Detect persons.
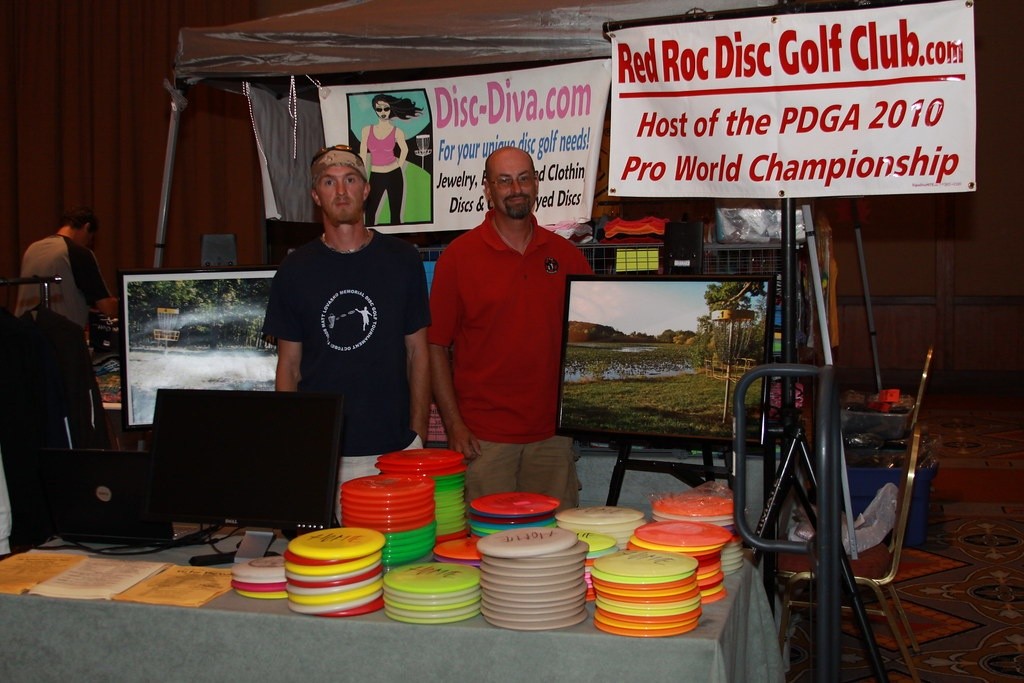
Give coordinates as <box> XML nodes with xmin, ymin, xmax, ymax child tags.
<box><xmin>14</xmin><ymin>205</ymin><xmax>118</xmax><ymax>331</ymax></box>
<box><xmin>431</xmin><ymin>146</ymin><xmax>596</xmax><ymax>508</ymax></box>
<box><xmin>263</xmin><ymin>145</ymin><xmax>432</xmax><ymax>523</ymax></box>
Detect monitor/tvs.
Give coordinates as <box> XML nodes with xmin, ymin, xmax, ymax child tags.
<box><xmin>149</xmin><ymin>389</ymin><xmax>344</xmax><ymax>566</ymax></box>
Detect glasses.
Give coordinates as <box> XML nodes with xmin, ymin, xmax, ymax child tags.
<box><xmin>311</xmin><ymin>145</ymin><xmax>362</xmax><ymax>164</ymax></box>
<box><xmin>489</xmin><ymin>174</ymin><xmax>535</xmax><ymax>186</ymax></box>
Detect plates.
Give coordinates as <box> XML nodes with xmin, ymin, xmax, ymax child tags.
<box><xmin>231</xmin><ymin>449</ymin><xmax>745</xmax><ymax>638</ymax></box>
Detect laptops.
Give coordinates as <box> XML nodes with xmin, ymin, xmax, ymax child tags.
<box><xmin>37</xmin><ymin>447</ymin><xmax>219</xmax><ymax>547</ymax></box>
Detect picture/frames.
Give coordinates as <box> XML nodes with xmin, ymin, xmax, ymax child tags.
<box><xmin>556</xmin><ymin>273</ymin><xmax>777</xmax><ymax>448</ymax></box>
<box><xmin>117</xmin><ymin>265</ymin><xmax>278</xmax><ymax>432</ymax></box>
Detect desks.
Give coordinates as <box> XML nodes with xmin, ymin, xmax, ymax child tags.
<box><xmin>0</xmin><ymin>521</ymin><xmax>800</xmax><ymax>683</ymax></box>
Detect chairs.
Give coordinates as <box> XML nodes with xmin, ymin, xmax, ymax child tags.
<box><xmin>776</xmin><ymin>423</ymin><xmax>921</xmax><ymax>683</ymax></box>
<box><xmin>887</xmin><ymin>345</ymin><xmax>934</xmax><ymax>446</ymax></box>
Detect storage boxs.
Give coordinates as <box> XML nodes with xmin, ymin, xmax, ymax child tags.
<box><xmin>714</xmin><ymin>197</ymin><xmax>806</xmax><ymax>243</ymax></box>
<box><xmin>845</xmin><ymin>449</ymin><xmax>935</xmax><ymax>547</ymax></box>
<box><xmin>840</xmin><ymin>394</ymin><xmax>915</xmax><ymax>439</ymax></box>
